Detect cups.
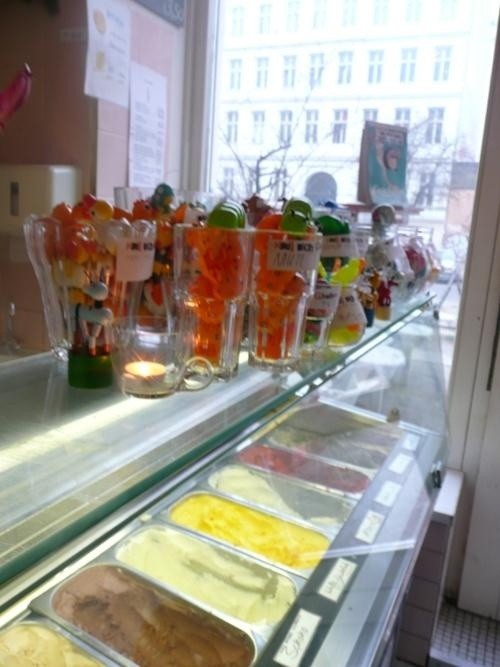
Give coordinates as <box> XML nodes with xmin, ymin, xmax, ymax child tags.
<box><xmin>20</xmin><ymin>214</ymin><xmax>367</xmax><ymax>399</ymax></box>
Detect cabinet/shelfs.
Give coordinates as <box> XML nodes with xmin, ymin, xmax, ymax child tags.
<box><xmin>0</xmin><ymin>289</ymin><xmax>453</xmax><ymax>667</ymax></box>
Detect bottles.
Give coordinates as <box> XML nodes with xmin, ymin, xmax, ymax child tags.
<box><xmin>353</xmin><ymin>223</ymin><xmax>443</xmax><ymax>308</ymax></box>
